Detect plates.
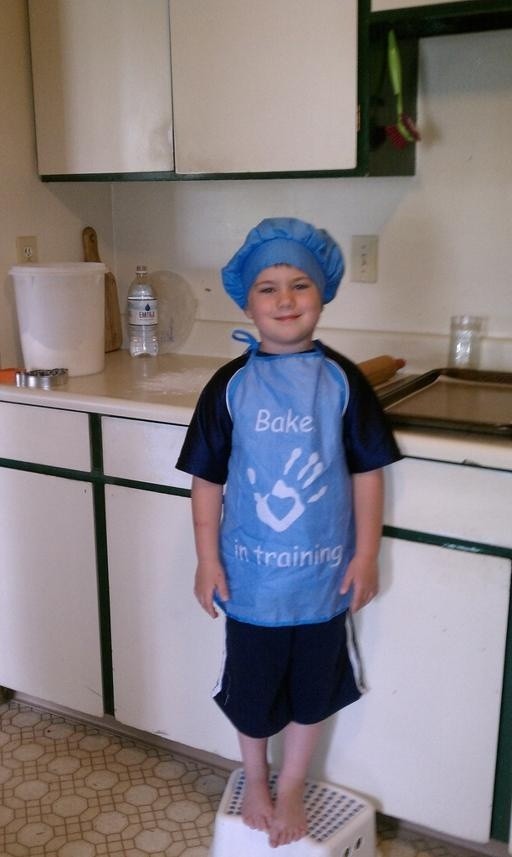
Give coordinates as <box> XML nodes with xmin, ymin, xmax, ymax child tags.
<box><xmin>379</xmin><ymin>366</ymin><xmax>511</xmax><ymax>438</ymax></box>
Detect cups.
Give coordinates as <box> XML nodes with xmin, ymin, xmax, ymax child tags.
<box><xmin>449</xmin><ymin>315</ymin><xmax>484</xmax><ymax>372</ymax></box>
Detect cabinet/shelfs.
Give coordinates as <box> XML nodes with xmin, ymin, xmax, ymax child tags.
<box><xmin>91</xmin><ymin>414</ymin><xmax>510</xmax><ymax>856</ymax></box>
<box><xmin>0</xmin><ymin>398</ymin><xmax>106</xmax><ymax>731</ymax></box>
<box><xmin>22</xmin><ymin>0</ymin><xmax>416</xmax><ymax>183</ymax></box>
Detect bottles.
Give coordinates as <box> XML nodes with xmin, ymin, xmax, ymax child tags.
<box><xmin>126</xmin><ymin>265</ymin><xmax>159</xmax><ymax>360</ymax></box>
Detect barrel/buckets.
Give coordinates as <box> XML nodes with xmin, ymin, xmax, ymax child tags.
<box><xmin>7</xmin><ymin>260</ymin><xmax>110</xmax><ymax>377</ymax></box>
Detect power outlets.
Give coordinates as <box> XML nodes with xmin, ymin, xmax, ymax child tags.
<box><xmin>349</xmin><ymin>234</ymin><xmax>378</xmax><ymax>285</ymax></box>
<box><xmin>15</xmin><ymin>235</ymin><xmax>39</xmax><ymax>266</ymax></box>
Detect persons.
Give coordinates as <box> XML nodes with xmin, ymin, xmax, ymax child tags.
<box><xmin>175</xmin><ymin>215</ymin><xmax>405</xmax><ymax>847</ymax></box>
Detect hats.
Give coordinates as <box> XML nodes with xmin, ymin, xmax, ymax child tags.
<box><xmin>219</xmin><ymin>216</ymin><xmax>347</xmax><ymax>311</ymax></box>
